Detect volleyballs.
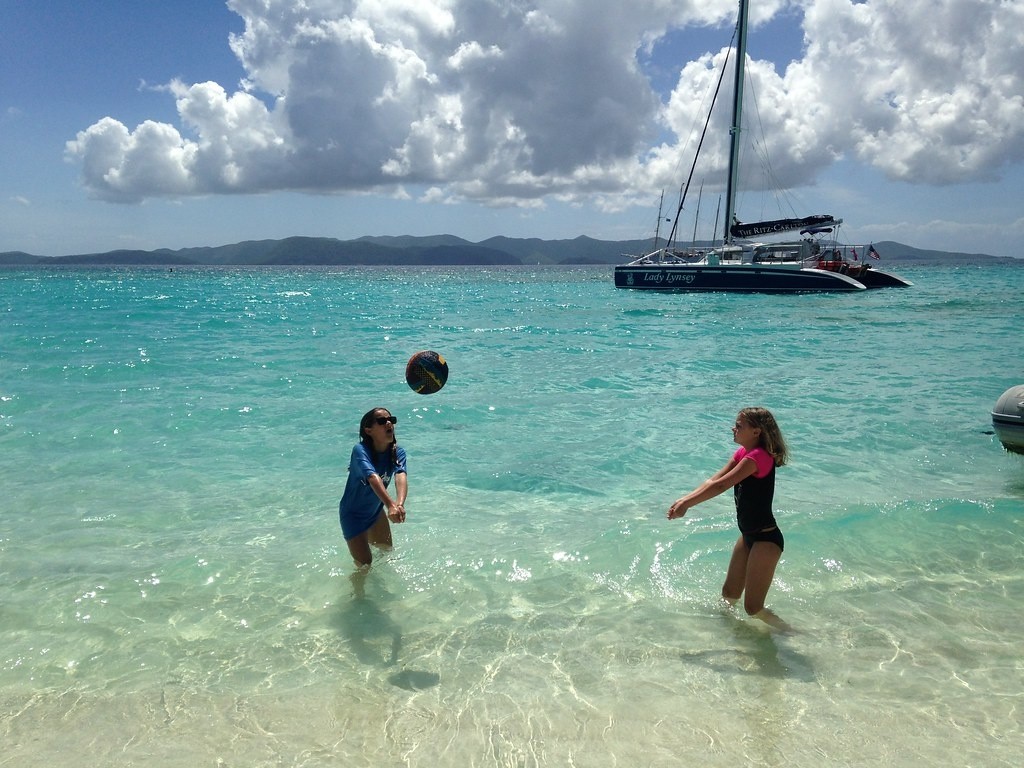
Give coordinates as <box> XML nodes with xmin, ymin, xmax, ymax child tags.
<box><xmin>404</xmin><ymin>350</ymin><xmax>449</xmax><ymax>395</ymax></box>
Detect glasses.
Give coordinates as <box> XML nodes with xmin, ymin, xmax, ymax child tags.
<box><xmin>375</xmin><ymin>417</ymin><xmax>396</xmax><ymax>425</ymax></box>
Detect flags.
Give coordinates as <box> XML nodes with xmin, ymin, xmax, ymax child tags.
<box><xmin>866</xmin><ymin>243</ymin><xmax>880</xmax><ymax>260</ymax></box>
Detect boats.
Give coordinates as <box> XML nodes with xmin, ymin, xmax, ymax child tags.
<box><xmin>817</xmin><ymin>261</ymin><xmax>871</xmax><ymax>277</ymax></box>
<box><xmin>992</xmin><ymin>385</ymin><xmax>1024</xmax><ymax>455</ymax></box>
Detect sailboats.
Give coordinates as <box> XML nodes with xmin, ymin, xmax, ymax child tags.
<box><xmin>613</xmin><ymin>1</ymin><xmax>914</xmax><ymax>293</ymax></box>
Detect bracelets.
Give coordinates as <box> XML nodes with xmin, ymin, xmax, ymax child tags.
<box><xmin>397</xmin><ymin>505</ymin><xmax>404</xmax><ymax>508</ymax></box>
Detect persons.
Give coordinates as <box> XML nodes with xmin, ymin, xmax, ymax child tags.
<box><xmin>666</xmin><ymin>407</ymin><xmax>790</xmax><ymax>615</ymax></box>
<box><xmin>850</xmin><ymin>249</ymin><xmax>858</xmax><ymax>261</ymax></box>
<box><xmin>339</xmin><ymin>409</ymin><xmax>408</xmax><ymax>565</ymax></box>
